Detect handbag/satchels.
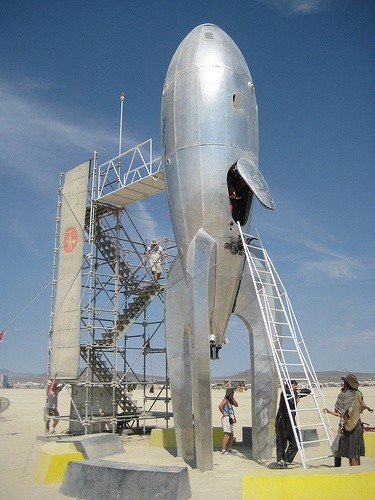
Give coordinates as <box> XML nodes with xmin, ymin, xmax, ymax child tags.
<box><xmin>229</xmin><ymin>414</ymin><xmax>236</xmax><ymax>423</ymax></box>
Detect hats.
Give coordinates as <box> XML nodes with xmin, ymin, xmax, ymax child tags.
<box><xmin>285</xmin><ymin>380</ymin><xmax>297</xmax><ymax>387</ymax></box>
<box><xmin>341</xmin><ymin>374</ymin><xmax>359</xmax><ymax>388</ymax></box>
<box><xmin>225</xmin><ymin>387</ymin><xmax>237</xmax><ymax>397</ymax></box>
<box><xmin>150</xmin><ymin>239</ymin><xmax>159</xmax><ymax>244</ymax></box>
<box><xmin>47</xmin><ymin>380</ymin><xmax>58</xmax><ymax>386</ymax></box>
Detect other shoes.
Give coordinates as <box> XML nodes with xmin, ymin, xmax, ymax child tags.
<box><xmin>229</xmin><ymin>449</ymin><xmax>238</xmax><ymax>455</ymax></box>
<box><xmin>51</xmin><ymin>429</ymin><xmax>58</xmax><ymax>435</ymax></box>
<box><xmin>44</xmin><ymin>433</ymin><xmax>50</xmax><ymax>435</ymax></box>
<box><xmin>277</xmin><ymin>459</ymin><xmax>288</xmax><ymax>469</ymax></box>
<box><xmin>221</xmin><ymin>450</ymin><xmax>230</xmax><ymax>455</ymax></box>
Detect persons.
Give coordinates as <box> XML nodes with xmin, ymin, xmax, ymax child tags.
<box><xmin>218</xmin><ymin>389</ymin><xmax>238</xmax><ymax>455</ymax></box>
<box><xmin>44</xmin><ymin>372</ymin><xmax>65</xmax><ymax>435</ymax></box>
<box><xmin>147</xmin><ymin>240</ymin><xmax>164</xmax><ymax>283</ymax></box>
<box><xmin>275</xmin><ymin>380</ymin><xmax>312</xmax><ymax>469</ymax></box>
<box><xmin>323</xmin><ymin>374</ymin><xmax>366</xmax><ymax>467</ymax></box>
<box><xmin>228</xmin><ymin>177</ymin><xmax>246</xmax><ymax>214</ymax></box>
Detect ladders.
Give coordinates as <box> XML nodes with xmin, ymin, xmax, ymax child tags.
<box><xmin>237</xmin><ymin>219</ymin><xmax>335</xmax><ymax>472</ymax></box>
